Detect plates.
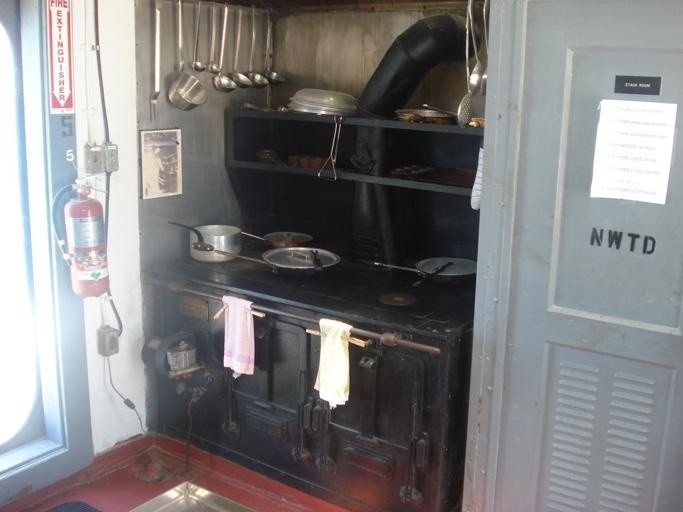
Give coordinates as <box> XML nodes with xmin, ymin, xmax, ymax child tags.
<box><xmin>394</xmin><ymin>109</ymin><xmax>458</xmax><ymax>125</ymax></box>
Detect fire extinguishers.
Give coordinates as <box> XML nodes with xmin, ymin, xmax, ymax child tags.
<box><xmin>51</xmin><ymin>177</ymin><xmax>110</xmax><ymax>298</ymax></box>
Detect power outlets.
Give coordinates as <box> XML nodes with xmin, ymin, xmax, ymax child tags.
<box><xmin>97</xmin><ymin>325</ymin><xmax>122</xmax><ymax>356</ymax></box>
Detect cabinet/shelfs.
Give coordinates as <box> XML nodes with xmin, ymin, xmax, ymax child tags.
<box><xmin>224</xmin><ymin>108</ymin><xmax>484</xmax><ymax>286</ymax></box>
<box><xmin>144</xmin><ymin>257</ymin><xmax>472</xmax><ymax>512</ymax></box>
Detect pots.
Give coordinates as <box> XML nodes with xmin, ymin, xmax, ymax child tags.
<box><xmin>374</xmin><ymin>256</ymin><xmax>477</xmax><ymax>282</ymax></box>
<box><xmin>241</xmin><ymin>231</ymin><xmax>314</xmax><ymax>250</ymax></box>
<box><xmin>168</xmin><ymin>221</ymin><xmax>243</xmax><ymax>264</ymax></box>
<box><xmin>215</xmin><ymin>246</ymin><xmax>342</xmax><ymax>275</ymax></box>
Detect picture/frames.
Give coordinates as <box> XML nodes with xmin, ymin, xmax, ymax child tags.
<box><xmin>140</xmin><ymin>128</ymin><xmax>183</xmax><ymax>200</ymax></box>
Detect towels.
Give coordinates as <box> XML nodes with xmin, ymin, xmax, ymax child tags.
<box><xmin>313</xmin><ymin>318</ymin><xmax>353</xmax><ymax>411</ymax></box>
<box><xmin>221</xmin><ymin>295</ymin><xmax>254</xmax><ymax>379</ymax></box>
